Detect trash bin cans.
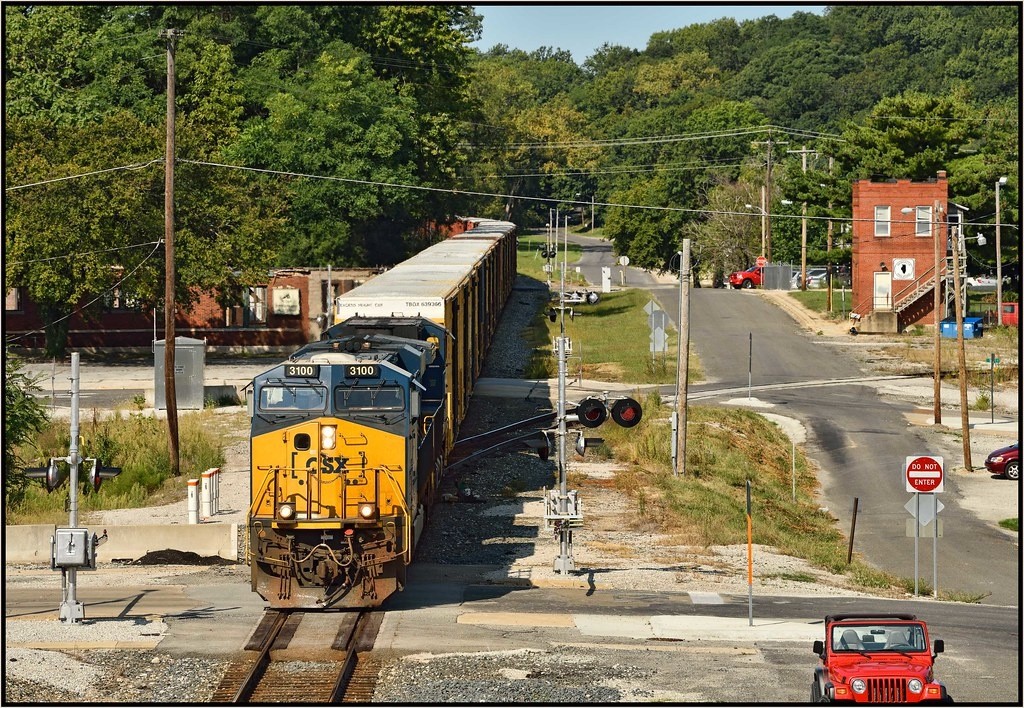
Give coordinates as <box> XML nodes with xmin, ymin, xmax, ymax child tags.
<box><xmin>940</xmin><ymin>316</ymin><xmax>983</xmax><ymax>339</ymax></box>
<box><xmin>601</xmin><ymin>266</ymin><xmax>611</xmax><ymax>293</ymax></box>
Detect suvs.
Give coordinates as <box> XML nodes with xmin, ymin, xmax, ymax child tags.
<box><xmin>985</xmin><ymin>303</ymin><xmax>1018</xmax><ymax>329</ymax></box>
<box><xmin>810</xmin><ymin>612</ymin><xmax>954</xmax><ymax>704</ymax></box>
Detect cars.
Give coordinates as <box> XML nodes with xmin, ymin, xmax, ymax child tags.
<box><xmin>985</xmin><ymin>444</ymin><xmax>1019</xmax><ymax>480</ymax></box>
<box><xmin>731</xmin><ymin>264</ymin><xmax>852</xmax><ymax>289</ymax></box>
<box><xmin>967</xmin><ymin>275</ymin><xmax>1011</xmax><ymax>286</ymax></box>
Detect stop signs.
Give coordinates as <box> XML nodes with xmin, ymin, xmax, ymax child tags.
<box><xmin>756</xmin><ymin>255</ymin><xmax>768</xmax><ymax>266</ymax></box>
<box><xmin>905</xmin><ymin>456</ymin><xmax>943</xmax><ymax>493</ymax></box>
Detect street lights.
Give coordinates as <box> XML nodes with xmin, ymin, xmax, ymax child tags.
<box><xmin>952</xmin><ymin>226</ymin><xmax>987</xmax><ymax>471</ymax></box>
<box><xmin>564</xmin><ymin>215</ymin><xmax>571</xmax><ymax>278</ymax></box>
<box><xmin>746</xmin><ymin>186</ymin><xmax>766</xmax><ymax>256</ymax></box>
<box><xmin>900</xmin><ymin>200</ymin><xmax>942</xmax><ymax>425</ymax></box>
<box><xmin>996</xmin><ymin>177</ymin><xmax>1008</xmax><ymax>326</ymax></box>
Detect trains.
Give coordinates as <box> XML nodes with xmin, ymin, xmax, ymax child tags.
<box><xmin>240</xmin><ymin>215</ymin><xmax>517</xmax><ymax>608</ymax></box>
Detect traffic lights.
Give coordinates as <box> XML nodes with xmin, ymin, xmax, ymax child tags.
<box><xmin>575</xmin><ymin>397</ymin><xmax>643</xmax><ymax>428</ymax></box>
<box><xmin>26</xmin><ymin>458</ymin><xmax>58</xmax><ymax>493</ymax></box>
<box><xmin>88</xmin><ymin>459</ymin><xmax>122</xmax><ymax>493</ymax></box>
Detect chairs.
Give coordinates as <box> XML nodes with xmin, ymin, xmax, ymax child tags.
<box><xmin>836</xmin><ymin>630</ymin><xmax>865</xmax><ymax>650</ymax></box>
<box><xmin>883</xmin><ymin>631</ymin><xmax>910</xmax><ymax>651</ymax></box>
